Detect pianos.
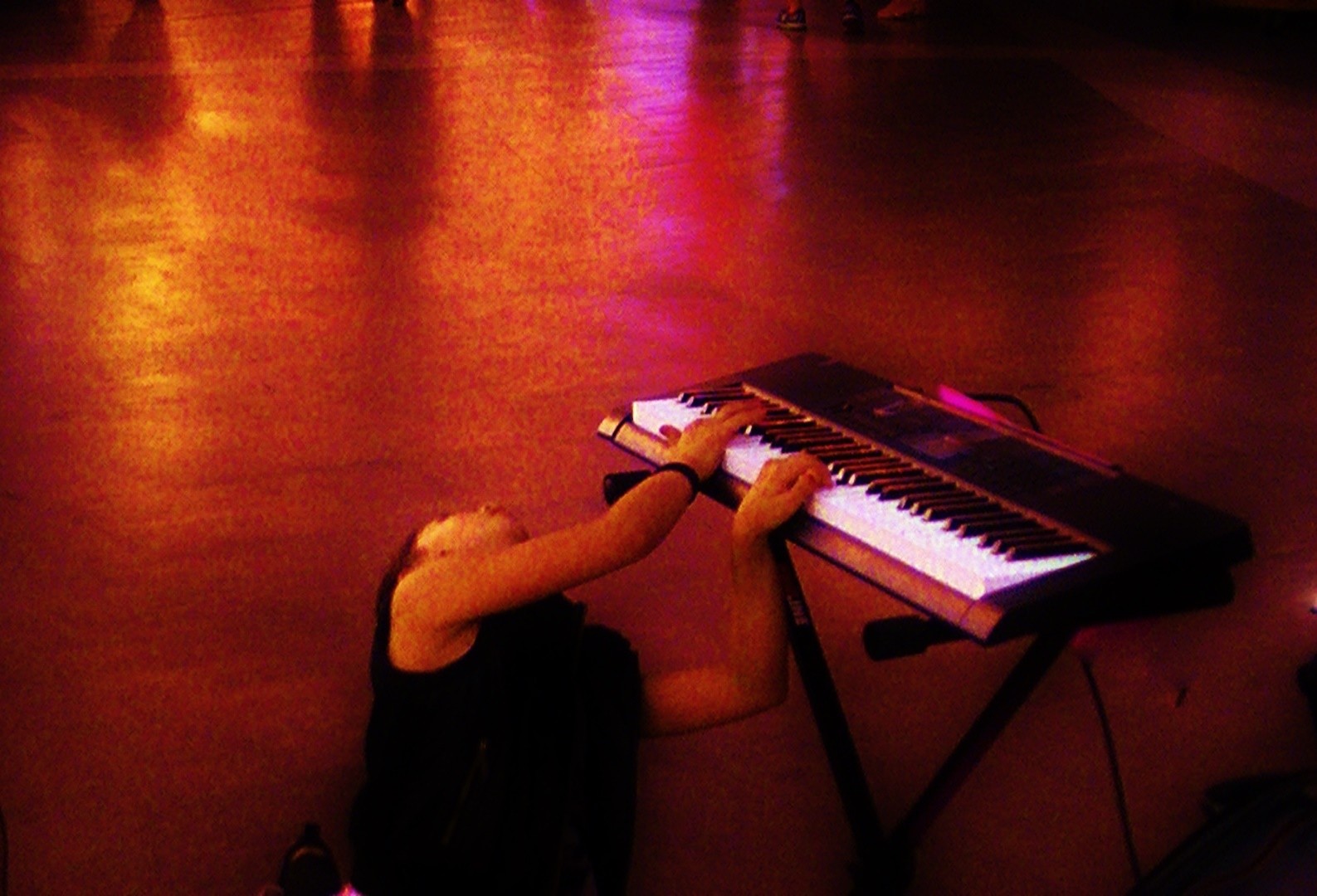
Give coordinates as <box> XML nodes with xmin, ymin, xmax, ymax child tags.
<box><xmin>594</xmin><ymin>350</ymin><xmax>1255</xmax><ymax>896</ymax></box>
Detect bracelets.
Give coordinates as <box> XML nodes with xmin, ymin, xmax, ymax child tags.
<box><xmin>652</xmin><ymin>462</ymin><xmax>700</xmax><ymax>504</ymax></box>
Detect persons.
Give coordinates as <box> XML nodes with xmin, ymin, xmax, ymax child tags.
<box><xmin>22</xmin><ymin>0</ymin><xmax>933</xmax><ymax>58</ymax></box>
<box><xmin>276</xmin><ymin>401</ymin><xmax>833</xmax><ymax>896</ymax></box>
